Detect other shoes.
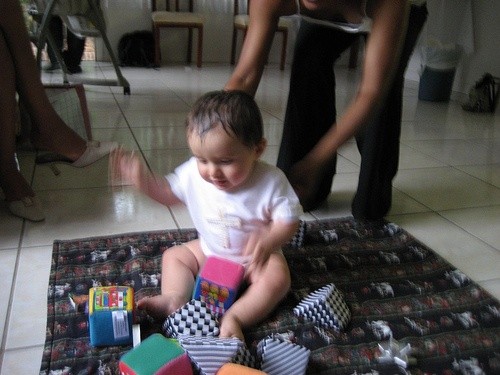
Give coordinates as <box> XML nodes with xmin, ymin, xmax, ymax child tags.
<box><xmin>41</xmin><ymin>62</ymin><xmax>60</xmax><ymax>72</ymax></box>
<box><xmin>353</xmin><ymin>181</ymin><xmax>393</xmax><ymax>221</ymax></box>
<box><xmin>67</xmin><ymin>62</ymin><xmax>81</xmax><ymax>74</ymax></box>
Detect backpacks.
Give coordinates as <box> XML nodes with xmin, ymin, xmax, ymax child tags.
<box><xmin>461</xmin><ymin>72</ymin><xmax>500</xmax><ymax>113</ymax></box>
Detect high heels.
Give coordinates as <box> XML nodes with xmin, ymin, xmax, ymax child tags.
<box><xmin>6</xmin><ymin>193</ymin><xmax>44</xmax><ymax>221</ymax></box>
<box><xmin>35</xmin><ymin>142</ymin><xmax>120</xmax><ymax>176</ymax></box>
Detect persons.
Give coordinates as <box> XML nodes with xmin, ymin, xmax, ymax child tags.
<box><xmin>224</xmin><ymin>0</ymin><xmax>428</xmax><ymax>222</ymax></box>
<box><xmin>0</xmin><ymin>1</ymin><xmax>119</xmax><ymax>222</ymax></box>
<box><xmin>115</xmin><ymin>88</ymin><xmax>304</xmax><ymax>346</ymax></box>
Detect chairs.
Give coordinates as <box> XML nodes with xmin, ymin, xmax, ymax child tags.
<box><xmin>151</xmin><ymin>0</ymin><xmax>204</xmax><ymax>68</ymax></box>
<box><xmin>231</xmin><ymin>0</ymin><xmax>290</xmax><ymax>71</ymax></box>
<box><xmin>27</xmin><ymin>0</ymin><xmax>131</xmax><ymax>95</ymax></box>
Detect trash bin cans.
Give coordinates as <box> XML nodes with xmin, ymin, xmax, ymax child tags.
<box><xmin>417</xmin><ymin>39</ymin><xmax>464</xmax><ymax>103</ymax></box>
